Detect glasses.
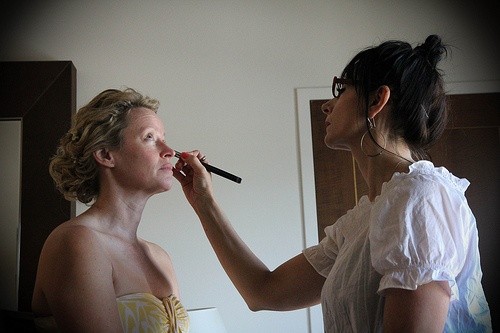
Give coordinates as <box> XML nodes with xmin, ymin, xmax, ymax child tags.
<box><xmin>332</xmin><ymin>76</ymin><xmax>351</xmax><ymax>98</ymax></box>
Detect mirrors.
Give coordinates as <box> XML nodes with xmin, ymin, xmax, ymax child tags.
<box><xmin>0</xmin><ymin>114</ymin><xmax>24</xmax><ymax>314</ymax></box>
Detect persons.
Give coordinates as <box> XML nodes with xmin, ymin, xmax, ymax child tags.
<box><xmin>29</xmin><ymin>87</ymin><xmax>191</xmax><ymax>333</ymax></box>
<box><xmin>173</xmin><ymin>34</ymin><xmax>497</xmax><ymax>332</ymax></box>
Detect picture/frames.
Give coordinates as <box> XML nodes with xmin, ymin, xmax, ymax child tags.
<box><xmin>295</xmin><ymin>79</ymin><xmax>499</xmax><ymax>332</ymax></box>
